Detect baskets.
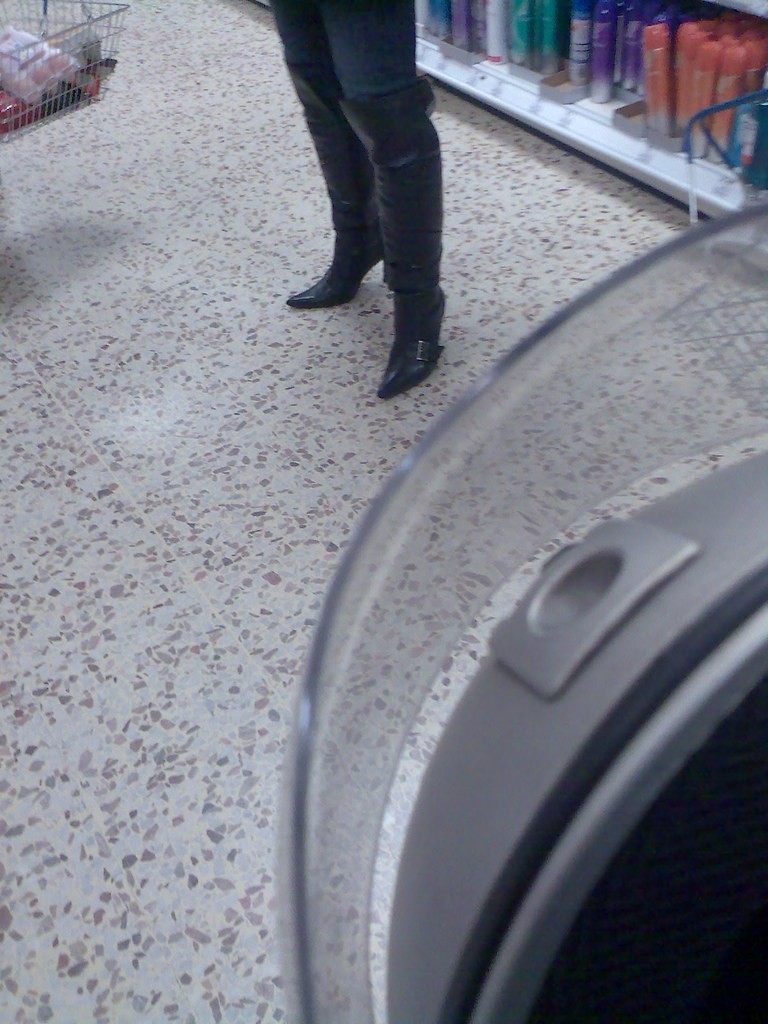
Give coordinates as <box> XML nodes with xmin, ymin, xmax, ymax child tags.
<box><xmin>662</xmin><ymin>234</ymin><xmax>768</xmax><ymax>420</ymax></box>
<box><xmin>0</xmin><ymin>0</ymin><xmax>131</xmax><ymax>143</ymax></box>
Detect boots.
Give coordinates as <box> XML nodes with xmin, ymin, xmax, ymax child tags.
<box><xmin>284</xmin><ymin>61</ymin><xmax>384</xmax><ymax>308</ymax></box>
<box><xmin>338</xmin><ymin>76</ymin><xmax>449</xmax><ymax>398</ymax></box>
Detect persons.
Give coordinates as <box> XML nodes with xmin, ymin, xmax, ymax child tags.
<box><xmin>276</xmin><ymin>1</ymin><xmax>451</xmax><ymax>399</ymax></box>
